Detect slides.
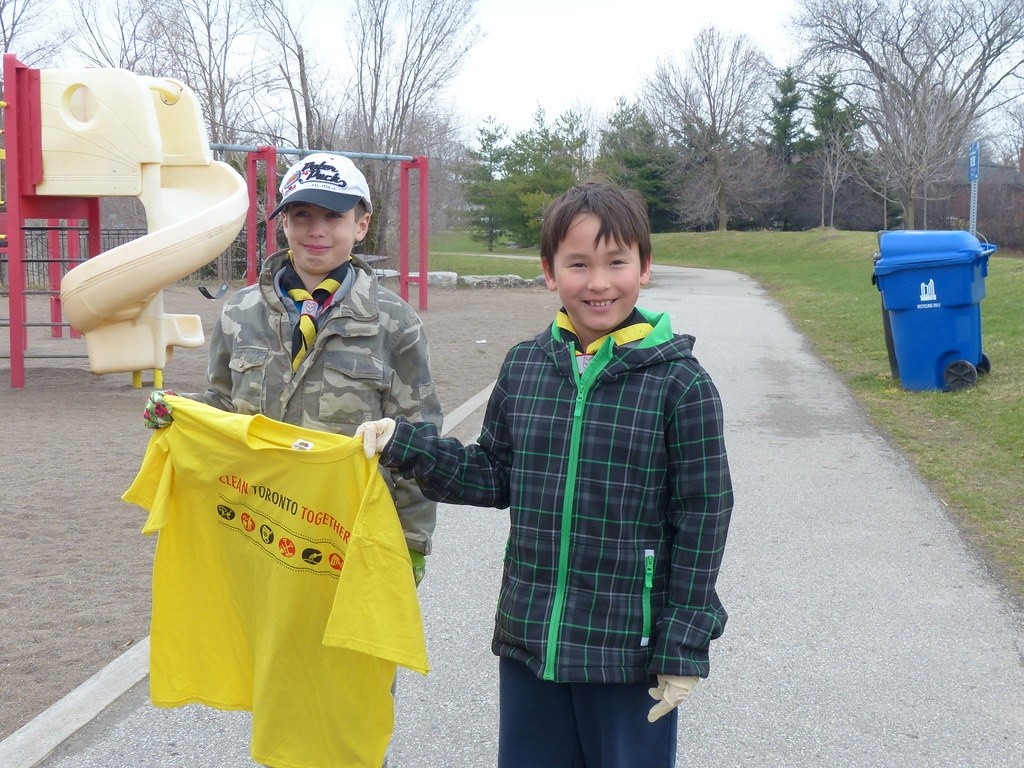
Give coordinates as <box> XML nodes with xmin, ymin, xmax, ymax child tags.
<box><xmin>58</xmin><ymin>158</ymin><xmax>252</xmax><ymax>378</ymax></box>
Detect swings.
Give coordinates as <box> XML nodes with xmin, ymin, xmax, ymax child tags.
<box><xmin>197</xmin><ymin>148</ymin><xmax>230</xmax><ymax>300</ymax></box>
<box><xmin>357</xmin><ymin>152</ymin><xmax>389</xmax><ymax>282</ymax></box>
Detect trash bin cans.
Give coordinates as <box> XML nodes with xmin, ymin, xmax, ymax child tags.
<box><xmin>871</xmin><ymin>228</ymin><xmax>998</xmax><ymax>394</ymax></box>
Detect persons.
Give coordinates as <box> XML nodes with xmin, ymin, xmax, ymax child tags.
<box><xmin>353</xmin><ymin>180</ymin><xmax>734</xmax><ymax>768</ymax></box>
<box><xmin>143</xmin><ymin>153</ymin><xmax>443</xmax><ymax>767</ymax></box>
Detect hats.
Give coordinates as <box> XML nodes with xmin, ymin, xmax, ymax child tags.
<box><xmin>268</xmin><ymin>153</ymin><xmax>372</xmax><ymax>222</ymax></box>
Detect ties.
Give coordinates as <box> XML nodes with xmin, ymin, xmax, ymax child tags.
<box><xmin>556</xmin><ymin>305</ymin><xmax>654</xmax><ymax>356</ymax></box>
<box><xmin>282</xmin><ymin>248</ymin><xmax>352</xmax><ymax>374</ymax></box>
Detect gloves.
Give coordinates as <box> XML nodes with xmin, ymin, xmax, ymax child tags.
<box><xmin>408</xmin><ymin>548</ymin><xmax>426</xmax><ymax>589</ymax></box>
<box><xmin>647</xmin><ymin>675</ymin><xmax>699</xmax><ymax>723</ymax></box>
<box><xmin>354</xmin><ymin>418</ymin><xmax>396</xmax><ymax>458</ymax></box>
<box><xmin>143</xmin><ymin>389</ymin><xmax>181</xmax><ymax>429</ymax></box>
